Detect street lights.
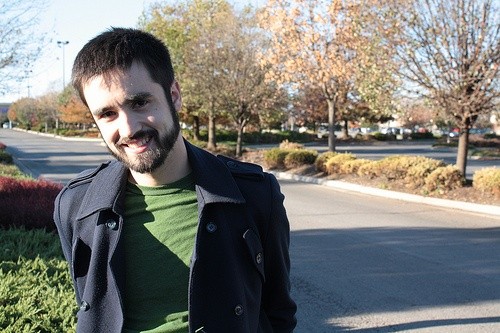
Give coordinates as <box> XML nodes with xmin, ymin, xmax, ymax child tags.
<box><xmin>57</xmin><ymin>41</ymin><xmax>70</xmax><ymax>90</ymax></box>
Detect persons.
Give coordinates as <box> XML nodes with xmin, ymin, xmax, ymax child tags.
<box><xmin>52</xmin><ymin>25</ymin><xmax>297</xmax><ymax>333</ymax></box>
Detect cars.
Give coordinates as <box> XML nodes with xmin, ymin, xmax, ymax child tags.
<box><xmin>298</xmin><ymin>122</ymin><xmax>495</xmax><ymax>139</ymax></box>
